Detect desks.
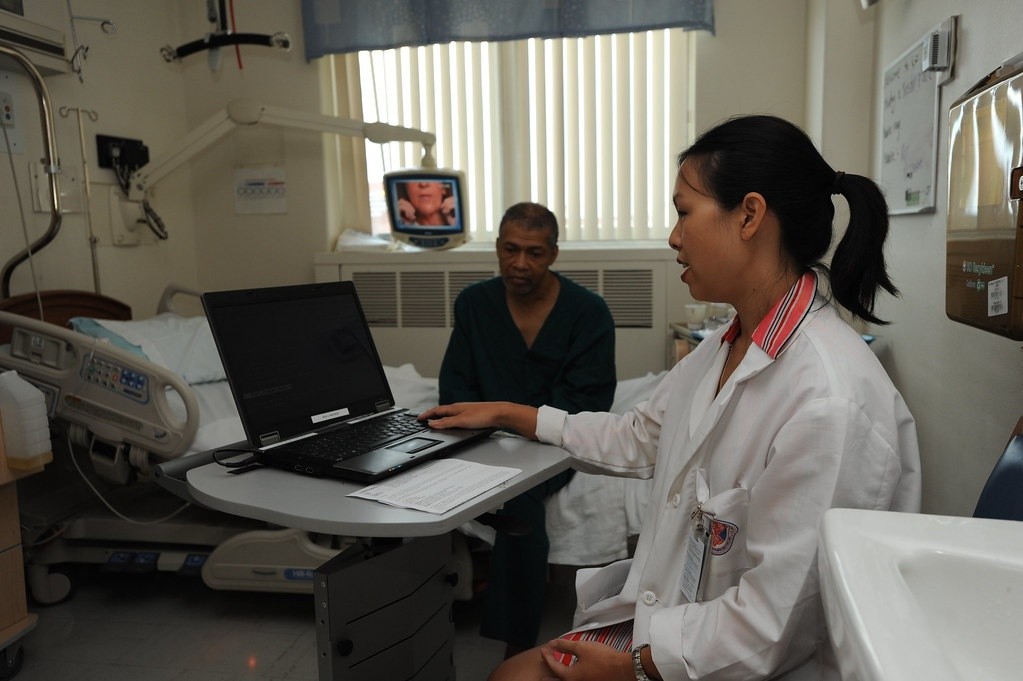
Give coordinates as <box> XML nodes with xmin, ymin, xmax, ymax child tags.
<box><xmin>151</xmin><ymin>430</ymin><xmax>572</xmax><ymax>536</ymax></box>
<box><xmin>670</xmin><ymin>322</ymin><xmax>877</xmax><ymax>363</ymax></box>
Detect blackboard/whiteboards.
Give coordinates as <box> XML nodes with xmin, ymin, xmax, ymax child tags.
<box><xmin>877</xmin><ymin>22</ymin><xmax>942</xmax><ymax>217</ymax></box>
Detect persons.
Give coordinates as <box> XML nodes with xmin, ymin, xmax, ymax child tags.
<box><xmin>439</xmin><ymin>202</ymin><xmax>619</xmax><ymax>663</ymax></box>
<box><xmin>396</xmin><ymin>182</ymin><xmax>455</xmax><ymax>226</ymax></box>
<box><xmin>417</xmin><ymin>115</ymin><xmax>922</xmax><ymax>681</ymax></box>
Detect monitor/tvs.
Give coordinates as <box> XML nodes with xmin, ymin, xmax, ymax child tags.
<box><xmin>384</xmin><ymin>170</ymin><xmax>470</xmax><ymax>250</ymax></box>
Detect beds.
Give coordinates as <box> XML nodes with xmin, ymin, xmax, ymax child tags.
<box><xmin>0</xmin><ymin>283</ymin><xmax>666</xmax><ymax>547</ymax></box>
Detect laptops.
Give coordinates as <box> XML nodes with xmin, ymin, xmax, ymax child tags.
<box><xmin>201</xmin><ymin>280</ymin><xmax>495</xmax><ymax>484</ymax></box>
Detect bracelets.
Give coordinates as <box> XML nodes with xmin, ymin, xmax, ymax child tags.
<box><xmin>631</xmin><ymin>644</ymin><xmax>651</xmax><ymax>681</ymax></box>
